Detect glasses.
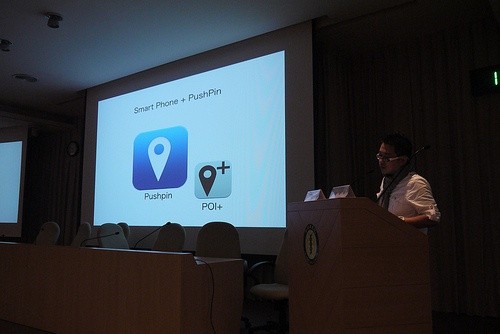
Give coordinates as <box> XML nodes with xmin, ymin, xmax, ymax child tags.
<box><xmin>376</xmin><ymin>152</ymin><xmax>409</xmax><ymax>162</ymax></box>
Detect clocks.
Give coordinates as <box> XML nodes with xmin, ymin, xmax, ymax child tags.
<box><xmin>66</xmin><ymin>141</ymin><xmax>79</xmax><ymax>157</ymax></box>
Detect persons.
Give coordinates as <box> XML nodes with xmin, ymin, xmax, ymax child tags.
<box><xmin>373</xmin><ymin>133</ymin><xmax>441</xmax><ymax>238</ymax></box>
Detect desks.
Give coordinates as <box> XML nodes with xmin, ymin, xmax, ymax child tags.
<box><xmin>0</xmin><ymin>242</ymin><xmax>244</xmax><ymax>334</ymax></box>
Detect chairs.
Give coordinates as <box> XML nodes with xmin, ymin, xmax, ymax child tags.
<box><xmin>34</xmin><ymin>221</ymin><xmax>289</xmax><ymax>334</ymax></box>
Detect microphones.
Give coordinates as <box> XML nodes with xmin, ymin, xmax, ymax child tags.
<box><xmin>375</xmin><ymin>144</ymin><xmax>431</xmax><ymax>203</ymax></box>
<box><xmin>134</xmin><ymin>222</ymin><xmax>170</xmax><ymax>249</ymax></box>
<box><xmin>80</xmin><ymin>232</ymin><xmax>119</xmax><ymax>247</ymax></box>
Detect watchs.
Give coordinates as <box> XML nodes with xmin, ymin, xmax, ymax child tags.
<box><xmin>397</xmin><ymin>215</ymin><xmax>405</xmax><ymax>222</ymax></box>
